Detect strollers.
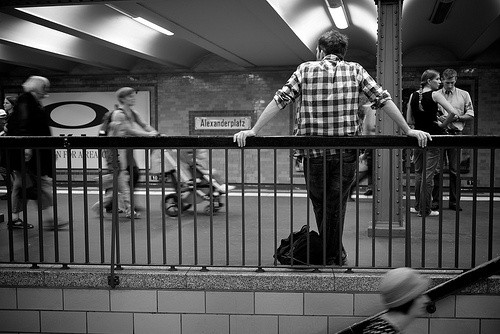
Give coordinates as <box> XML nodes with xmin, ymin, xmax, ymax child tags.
<box><xmin>148</xmin><ymin>134</ymin><xmax>227</xmax><ymax>217</ymax></box>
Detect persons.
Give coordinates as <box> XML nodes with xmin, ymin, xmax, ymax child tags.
<box><xmin>437</xmin><ymin>70</ymin><xmax>475</xmax><ymax>213</ymax></box>
<box><xmin>360</xmin><ymin>267</ymin><xmax>430</xmax><ymax>334</ymax></box>
<box><xmin>232</xmin><ymin>31</ymin><xmax>432</xmax><ymax>264</ymax></box>
<box><xmin>0</xmin><ymin>75</ymin><xmax>69</xmax><ymax>229</ymax></box>
<box><xmin>0</xmin><ymin>94</ymin><xmax>17</xmax><ymax>137</ymax></box>
<box><xmin>346</xmin><ymin>101</ymin><xmax>375</xmax><ymax>202</ymax></box>
<box><xmin>190</xmin><ymin>148</ymin><xmax>235</xmax><ymax>193</ymax></box>
<box><xmin>91</xmin><ymin>87</ymin><xmax>164</xmax><ymax>221</ymax></box>
<box><xmin>407</xmin><ymin>69</ymin><xmax>456</xmax><ymax>217</ymax></box>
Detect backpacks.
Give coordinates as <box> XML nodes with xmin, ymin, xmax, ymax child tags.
<box><xmin>273</xmin><ymin>225</ymin><xmax>323</xmax><ymax>265</ymax></box>
<box><xmin>99</xmin><ymin>104</ymin><xmax>137</xmax><ymax>136</ymax></box>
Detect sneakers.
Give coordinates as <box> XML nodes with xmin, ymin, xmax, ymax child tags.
<box><xmin>410</xmin><ymin>208</ymin><xmax>418</xmax><ymax>212</ymax></box>
<box><xmin>43</xmin><ymin>219</ymin><xmax>68</xmax><ymax>230</ymax></box>
<box><xmin>106</xmin><ymin>208</ymin><xmax>123</xmax><ymax>213</ymax></box>
<box><xmin>7</xmin><ymin>218</ymin><xmax>34</xmax><ymax>229</ymax></box>
<box><xmin>418</xmin><ymin>210</ymin><xmax>439</xmax><ymax>216</ymax></box>
<box><xmin>0</xmin><ymin>193</ymin><xmax>9</xmax><ymax>200</ymax></box>
<box><xmin>91</xmin><ymin>203</ymin><xmax>104</xmax><ymax>218</ymax></box>
<box><xmin>126</xmin><ymin>211</ymin><xmax>141</xmax><ymax>219</ymax></box>
<box><xmin>449</xmin><ymin>206</ymin><xmax>462</xmax><ymax>211</ymax></box>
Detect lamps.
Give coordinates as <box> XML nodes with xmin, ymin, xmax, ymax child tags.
<box><xmin>106</xmin><ymin>4</ymin><xmax>174</xmax><ymax>37</ymax></box>
<box><xmin>325</xmin><ymin>1</ymin><xmax>352</xmax><ymax>30</ymax></box>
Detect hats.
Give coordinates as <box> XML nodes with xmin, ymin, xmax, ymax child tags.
<box><xmin>380</xmin><ymin>267</ymin><xmax>428</xmax><ymax>308</ymax></box>
